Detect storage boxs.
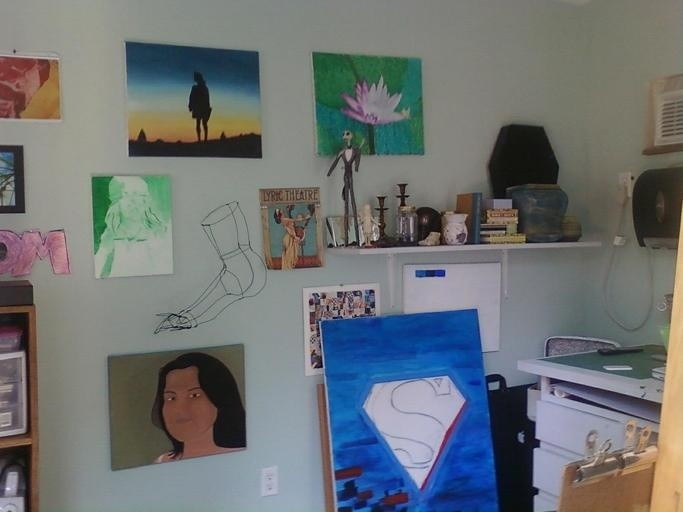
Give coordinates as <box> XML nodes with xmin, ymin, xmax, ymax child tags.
<box><xmin>1</xmin><ymin>279</ymin><xmax>34</xmax><ymax>307</ymax></box>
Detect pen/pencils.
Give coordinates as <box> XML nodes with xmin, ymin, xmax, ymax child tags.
<box><xmin>598</xmin><ymin>348</ymin><xmax>644</xmax><ymax>355</ymax></box>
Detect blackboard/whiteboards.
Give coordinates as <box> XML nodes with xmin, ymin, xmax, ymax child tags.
<box><xmin>402</xmin><ymin>262</ymin><xmax>502</xmax><ymax>354</ymax></box>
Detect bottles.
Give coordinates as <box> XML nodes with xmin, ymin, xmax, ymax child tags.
<box><xmin>397</xmin><ymin>206</ymin><xmax>417</xmax><ymax>245</ymax></box>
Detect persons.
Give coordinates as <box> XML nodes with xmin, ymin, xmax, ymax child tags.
<box><xmin>189</xmin><ymin>72</ymin><xmax>211</xmax><ymax>141</ymax></box>
<box><xmin>327</xmin><ymin>130</ymin><xmax>361</xmax><ymax>247</ymax></box>
<box><xmin>151</xmin><ymin>352</ymin><xmax>247</xmax><ymax>462</ymax></box>
<box><xmin>275</xmin><ymin>203</ymin><xmax>316</xmax><ymax>271</ymax></box>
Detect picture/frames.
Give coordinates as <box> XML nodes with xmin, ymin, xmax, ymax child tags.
<box><xmin>0</xmin><ymin>145</ymin><xmax>25</xmax><ymax>213</ymax></box>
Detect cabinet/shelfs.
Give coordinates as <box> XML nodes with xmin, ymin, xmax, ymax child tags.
<box><xmin>517</xmin><ymin>345</ymin><xmax>665</xmax><ymax>512</ymax></box>
<box><xmin>0</xmin><ymin>305</ymin><xmax>40</xmax><ymax>512</ymax></box>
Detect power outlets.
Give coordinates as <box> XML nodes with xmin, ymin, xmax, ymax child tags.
<box><xmin>618</xmin><ymin>172</ymin><xmax>632</xmax><ymax>198</ymax></box>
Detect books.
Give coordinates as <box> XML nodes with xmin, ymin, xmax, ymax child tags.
<box><xmin>456</xmin><ymin>193</ymin><xmax>526</xmax><ymax>243</ymax></box>
